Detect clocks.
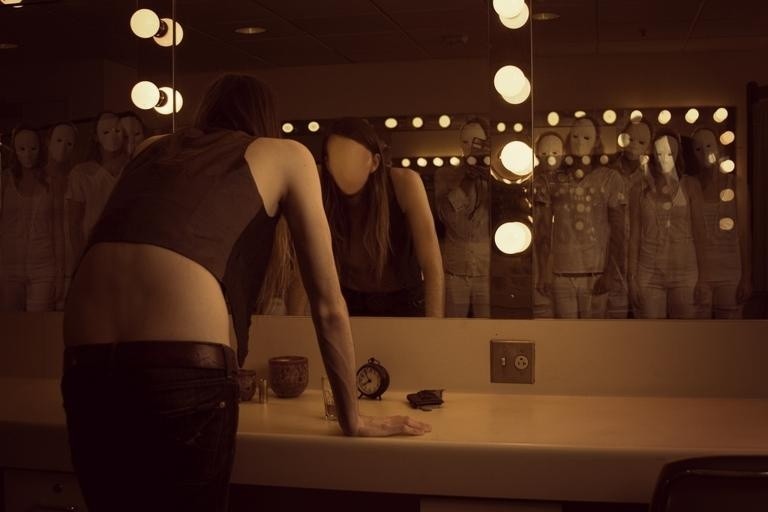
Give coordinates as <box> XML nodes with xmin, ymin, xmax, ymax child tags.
<box><xmin>356</xmin><ymin>357</ymin><xmax>390</xmax><ymax>400</ymax></box>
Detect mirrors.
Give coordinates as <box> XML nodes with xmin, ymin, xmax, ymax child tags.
<box><xmin>251</xmin><ymin>102</ymin><xmax>767</xmax><ymax>321</ymax></box>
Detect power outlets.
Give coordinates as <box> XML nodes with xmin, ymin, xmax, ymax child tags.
<box><xmin>489</xmin><ymin>338</ymin><xmax>536</xmax><ymax>385</ymax></box>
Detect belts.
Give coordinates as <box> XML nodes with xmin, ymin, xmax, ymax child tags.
<box><xmin>64</xmin><ymin>345</ymin><xmax>237</xmax><ymax>370</ymax></box>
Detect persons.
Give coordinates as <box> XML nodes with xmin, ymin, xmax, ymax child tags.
<box><xmin>1</xmin><ymin>109</ymin><xmax>753</xmax><ymax>320</ymax></box>
<box><xmin>60</xmin><ymin>75</ymin><xmax>432</xmax><ymax>512</ymax></box>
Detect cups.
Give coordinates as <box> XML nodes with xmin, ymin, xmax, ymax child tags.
<box><xmin>236</xmin><ymin>356</ymin><xmax>309</xmax><ymax>401</ymax></box>
<box><xmin>322</xmin><ymin>376</ymin><xmax>339</xmax><ymax>420</ymax></box>
